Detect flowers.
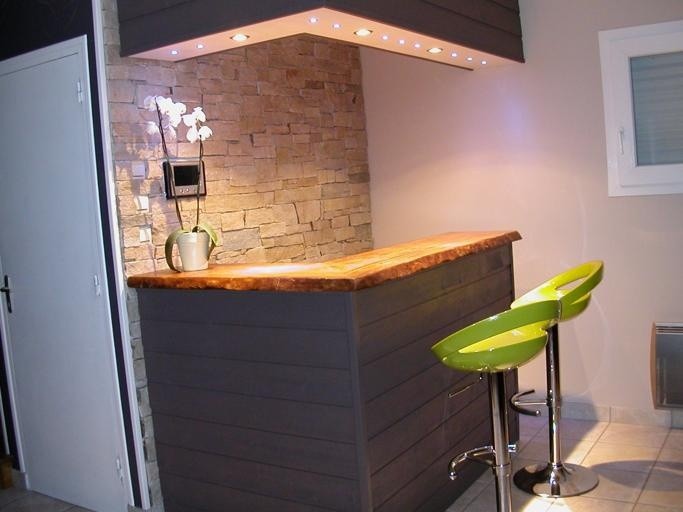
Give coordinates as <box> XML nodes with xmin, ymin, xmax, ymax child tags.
<box><xmin>142</xmin><ymin>95</ymin><xmax>222</xmax><ymax>275</ymax></box>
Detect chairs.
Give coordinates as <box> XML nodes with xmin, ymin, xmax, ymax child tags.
<box><xmin>510</xmin><ymin>259</ymin><xmax>606</xmax><ymax>498</ymax></box>
<box><xmin>432</xmin><ymin>299</ymin><xmax>563</xmax><ymax>512</ymax></box>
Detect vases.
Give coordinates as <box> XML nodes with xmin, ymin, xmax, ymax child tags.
<box><xmin>176</xmin><ymin>232</ymin><xmax>210</xmax><ymax>272</ymax></box>
